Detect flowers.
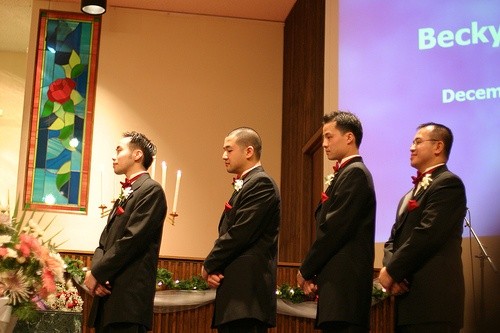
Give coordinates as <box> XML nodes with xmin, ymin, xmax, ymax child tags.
<box><xmin>324</xmin><ymin>173</ymin><xmax>336</xmax><ymax>186</ymax></box>
<box><xmin>232</xmin><ymin>176</ymin><xmax>243</xmax><ymax>192</ymax></box>
<box><xmin>119</xmin><ymin>178</ymin><xmax>134</xmax><ymax>201</ymax></box>
<box><xmin>419</xmin><ymin>173</ymin><xmax>433</xmax><ymax>189</ymax></box>
<box><xmin>0</xmin><ymin>210</ymin><xmax>83</xmax><ymax>333</ymax></box>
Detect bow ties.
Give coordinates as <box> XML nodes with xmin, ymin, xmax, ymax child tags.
<box><xmin>119</xmin><ymin>173</ymin><xmax>149</xmax><ymax>189</ymax></box>
<box><xmin>410</xmin><ymin>165</ymin><xmax>443</xmax><ymax>187</ymax></box>
<box><xmin>232</xmin><ymin>173</ymin><xmax>248</xmax><ymax>185</ymax></box>
<box><xmin>333</xmin><ymin>156</ymin><xmax>358</xmax><ymax>173</ymax></box>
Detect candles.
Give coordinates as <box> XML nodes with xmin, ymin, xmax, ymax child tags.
<box><xmin>151</xmin><ymin>154</ymin><xmax>157</xmax><ymax>180</ymax></box>
<box><xmin>172</xmin><ymin>171</ymin><xmax>182</xmax><ymax>213</ymax></box>
<box><xmin>161</xmin><ymin>161</ymin><xmax>167</xmax><ymax>189</ymax></box>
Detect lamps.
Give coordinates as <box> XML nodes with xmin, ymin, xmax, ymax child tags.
<box><xmin>81</xmin><ymin>0</ymin><xmax>106</xmax><ymax>15</ymax></box>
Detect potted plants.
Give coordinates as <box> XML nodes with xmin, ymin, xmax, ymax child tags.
<box><xmin>156</xmin><ymin>268</ymin><xmax>172</xmax><ymax>290</ymax></box>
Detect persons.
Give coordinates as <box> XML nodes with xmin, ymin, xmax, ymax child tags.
<box><xmin>202</xmin><ymin>126</ymin><xmax>281</xmax><ymax>333</ymax></box>
<box><xmin>297</xmin><ymin>111</ymin><xmax>377</xmax><ymax>333</ymax></box>
<box><xmin>378</xmin><ymin>122</ymin><xmax>466</xmax><ymax>333</ymax></box>
<box><xmin>83</xmin><ymin>130</ymin><xmax>166</xmax><ymax>333</ymax></box>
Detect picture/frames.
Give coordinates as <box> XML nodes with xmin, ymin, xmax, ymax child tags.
<box><xmin>23</xmin><ymin>9</ymin><xmax>101</xmax><ymax>215</ymax></box>
